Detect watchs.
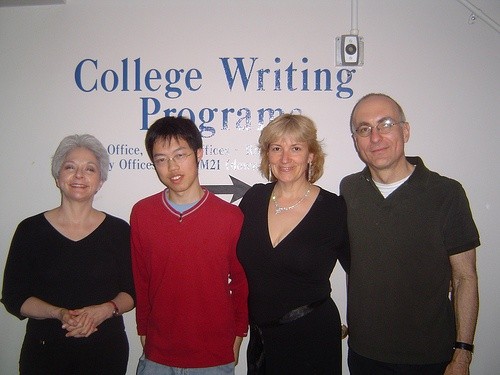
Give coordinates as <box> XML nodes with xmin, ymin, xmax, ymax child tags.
<box><xmin>106</xmin><ymin>301</ymin><xmax>119</xmax><ymax>320</ymax></box>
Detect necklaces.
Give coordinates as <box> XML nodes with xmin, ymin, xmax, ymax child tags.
<box><xmin>273</xmin><ymin>182</ymin><xmax>311</xmax><ymax>214</ymax></box>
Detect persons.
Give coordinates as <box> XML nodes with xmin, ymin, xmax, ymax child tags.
<box><xmin>339</xmin><ymin>94</ymin><xmax>480</xmax><ymax>375</ymax></box>
<box><xmin>130</xmin><ymin>117</ymin><xmax>249</xmax><ymax>375</ymax></box>
<box><xmin>237</xmin><ymin>115</ymin><xmax>351</xmax><ymax>375</ymax></box>
<box><xmin>0</xmin><ymin>135</ymin><xmax>135</xmax><ymax>375</ymax></box>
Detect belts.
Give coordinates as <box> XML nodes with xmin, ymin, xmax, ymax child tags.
<box><xmin>257</xmin><ymin>300</ymin><xmax>321</xmax><ymax>328</ymax></box>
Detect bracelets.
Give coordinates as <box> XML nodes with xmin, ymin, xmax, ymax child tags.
<box><xmin>453</xmin><ymin>342</ymin><xmax>474</xmax><ymax>354</ymax></box>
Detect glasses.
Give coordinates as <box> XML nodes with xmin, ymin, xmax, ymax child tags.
<box><xmin>353</xmin><ymin>120</ymin><xmax>404</xmax><ymax>137</ymax></box>
<box><xmin>150</xmin><ymin>149</ymin><xmax>196</xmax><ymax>167</ymax></box>
